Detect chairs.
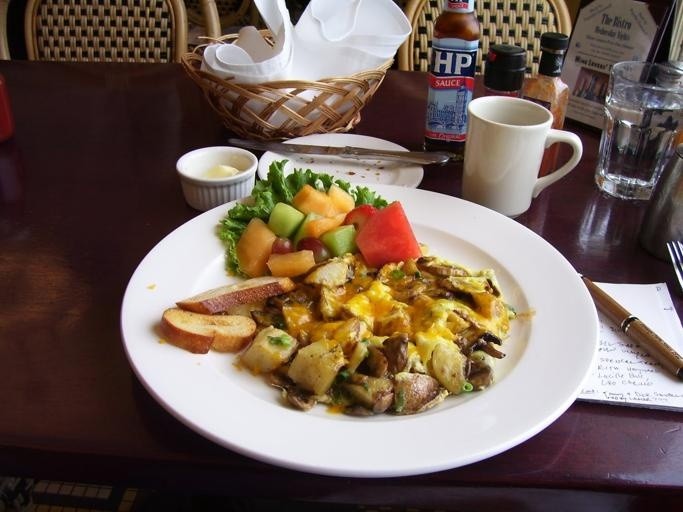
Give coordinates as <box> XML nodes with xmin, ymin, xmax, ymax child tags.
<box><xmin>397</xmin><ymin>0</ymin><xmax>573</xmax><ymax>73</ymax></box>
<box><xmin>0</xmin><ymin>2</ymin><xmax>222</xmax><ymax>66</ymax></box>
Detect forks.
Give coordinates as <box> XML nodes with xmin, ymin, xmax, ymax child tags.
<box><xmin>665</xmin><ymin>239</ymin><xmax>683</xmax><ymax>289</ymax></box>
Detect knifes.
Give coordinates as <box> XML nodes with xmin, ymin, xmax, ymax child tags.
<box><xmin>228</xmin><ymin>135</ymin><xmax>454</xmax><ymax>166</ymax></box>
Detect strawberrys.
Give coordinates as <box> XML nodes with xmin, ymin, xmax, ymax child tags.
<box><xmin>344</xmin><ymin>205</ymin><xmax>378</xmax><ymax>235</ymax></box>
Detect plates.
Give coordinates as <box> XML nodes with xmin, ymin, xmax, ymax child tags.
<box><xmin>258</xmin><ymin>132</ymin><xmax>425</xmax><ymax>188</ymax></box>
<box><xmin>120</xmin><ymin>183</ymin><xmax>599</xmax><ymax>476</ymax></box>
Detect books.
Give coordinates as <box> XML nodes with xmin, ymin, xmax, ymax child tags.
<box><xmin>577</xmin><ymin>282</ymin><xmax>683</xmax><ymax>411</ymax></box>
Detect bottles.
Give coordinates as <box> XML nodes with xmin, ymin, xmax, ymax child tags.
<box><xmin>425</xmin><ymin>0</ymin><xmax>568</xmax><ymax>158</ymax></box>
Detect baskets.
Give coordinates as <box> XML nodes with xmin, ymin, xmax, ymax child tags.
<box><xmin>180</xmin><ymin>28</ymin><xmax>395</xmax><ymax>142</ymax></box>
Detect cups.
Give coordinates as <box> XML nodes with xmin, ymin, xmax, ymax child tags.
<box><xmin>175</xmin><ymin>147</ymin><xmax>257</xmax><ymax>214</ymax></box>
<box><xmin>462</xmin><ymin>96</ymin><xmax>583</xmax><ymax>221</ymax></box>
<box><xmin>594</xmin><ymin>61</ymin><xmax>683</xmax><ymax>262</ymax></box>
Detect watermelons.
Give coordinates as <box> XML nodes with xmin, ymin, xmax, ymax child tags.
<box><xmin>356</xmin><ymin>201</ymin><xmax>422</xmax><ymax>265</ymax></box>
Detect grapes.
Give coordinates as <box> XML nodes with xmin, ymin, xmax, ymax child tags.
<box><xmin>272</xmin><ymin>237</ymin><xmax>292</xmax><ymax>257</ymax></box>
<box><xmin>297</xmin><ymin>236</ymin><xmax>331</xmax><ymax>261</ymax></box>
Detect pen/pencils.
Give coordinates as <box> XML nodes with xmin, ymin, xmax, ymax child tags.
<box><xmin>575</xmin><ymin>266</ymin><xmax>683</xmax><ymax>383</ymax></box>
